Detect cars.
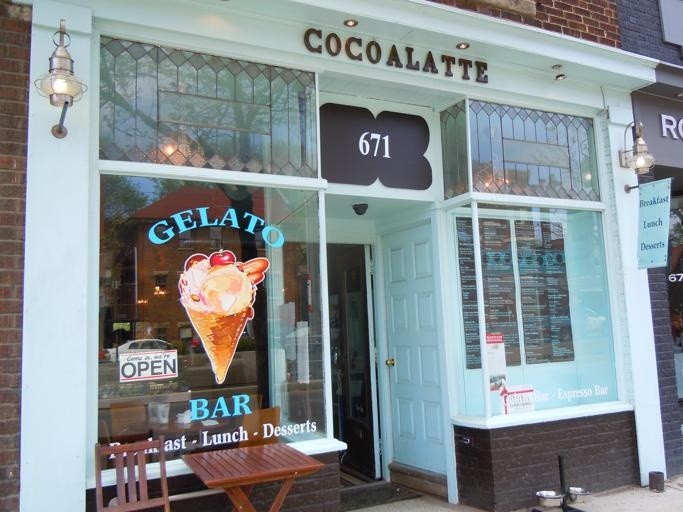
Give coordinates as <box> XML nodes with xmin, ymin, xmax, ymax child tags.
<box><xmin>105</xmin><ymin>339</ymin><xmax>204</xmax><ymax>369</ymax></box>
<box><xmin>191</xmin><ymin>331</ymin><xmax>248</xmax><ymax>353</ymax></box>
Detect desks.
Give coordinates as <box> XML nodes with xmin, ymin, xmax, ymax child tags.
<box><xmin>180</xmin><ymin>442</ymin><xmax>326</xmax><ymax>511</ymax></box>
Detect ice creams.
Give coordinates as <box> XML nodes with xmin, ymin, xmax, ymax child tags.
<box><xmin>177</xmin><ymin>248</ymin><xmax>269</xmax><ymax>385</ymax></box>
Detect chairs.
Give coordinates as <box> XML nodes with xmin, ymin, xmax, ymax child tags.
<box><xmin>92</xmin><ymin>398</ymin><xmax>172</xmax><ymax>511</ymax></box>
<box><xmin>236</xmin><ymin>406</ymin><xmax>281</xmax><ymax>448</ymax></box>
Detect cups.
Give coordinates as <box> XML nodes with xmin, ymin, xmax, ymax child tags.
<box><xmin>149</xmin><ymin>403</ymin><xmax>170</xmax><ymax>425</ymax></box>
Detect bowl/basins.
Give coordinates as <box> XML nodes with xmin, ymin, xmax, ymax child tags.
<box><xmin>536</xmin><ymin>490</ymin><xmax>565</xmax><ymax>507</ymax></box>
<box><xmin>569</xmin><ymin>487</ymin><xmax>592</xmax><ymax>502</ymax></box>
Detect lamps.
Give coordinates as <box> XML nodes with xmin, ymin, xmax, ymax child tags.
<box><xmin>32</xmin><ymin>20</ymin><xmax>87</xmax><ymax>138</ymax></box>
<box><xmin>618</xmin><ymin>119</ymin><xmax>656</xmax><ymax>176</ymax></box>
